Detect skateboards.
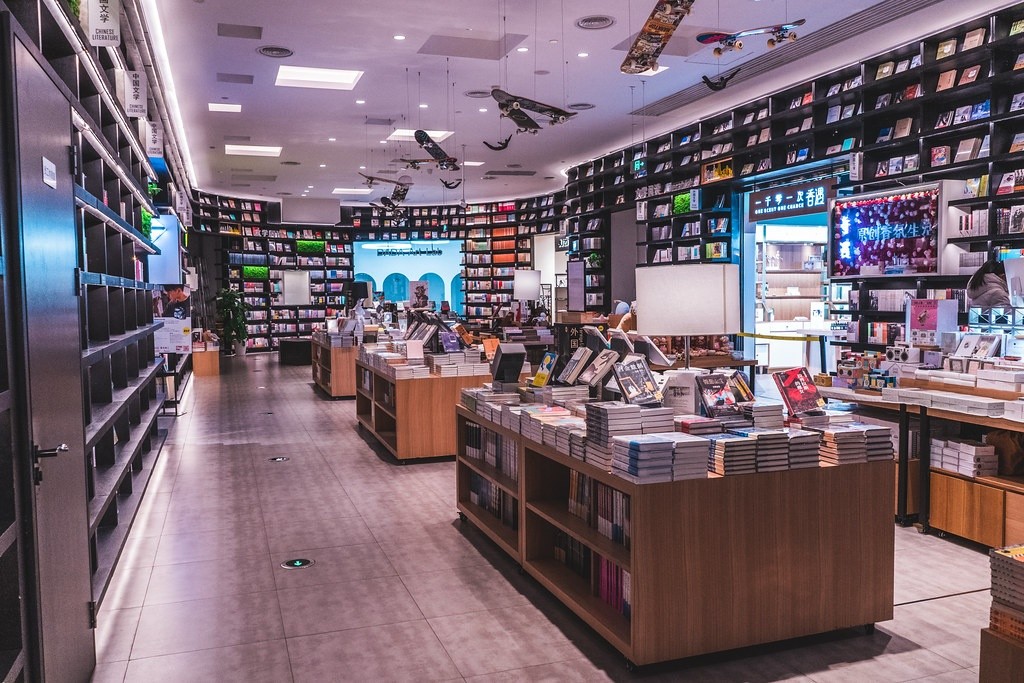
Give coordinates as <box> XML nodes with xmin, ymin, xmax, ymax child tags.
<box><xmin>619</xmin><ymin>0</ymin><xmax>696</xmax><ymax>76</ymax></box>
<box><xmin>413</xmin><ymin>129</ymin><xmax>461</xmax><ymax>172</ymax></box>
<box><xmin>695</xmin><ymin>17</ymin><xmax>808</xmax><ymax>60</ymax></box>
<box><xmin>357</xmin><ymin>170</ymin><xmax>415</xmax><ymax>189</ymax></box>
<box><xmin>368</xmin><ymin>175</ymin><xmax>413</xmax><ymax>222</ymax></box>
<box><xmin>490</xmin><ymin>88</ymin><xmax>579</xmax><ymax>137</ymax></box>
<box><xmin>399</xmin><ymin>156</ymin><xmax>458</xmax><ymax>171</ymax></box>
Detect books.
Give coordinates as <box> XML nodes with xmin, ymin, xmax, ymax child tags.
<box><xmin>195</xmin><ymin>15</ymin><xmax>1024</xmax><ymax>644</ymax></box>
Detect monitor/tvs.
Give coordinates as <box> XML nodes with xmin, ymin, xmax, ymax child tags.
<box><xmin>1003</xmin><ymin>257</ymin><xmax>1024</xmax><ymax>307</ymax></box>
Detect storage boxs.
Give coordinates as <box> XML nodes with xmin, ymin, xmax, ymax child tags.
<box><xmin>931</xmin><ymin>437</ymin><xmax>956</xmax><ymax>448</ymax></box>
<box><xmin>930</xmin><ymin>453</ymin><xmax>943</xmax><ymax>462</ymax></box>
<box><xmin>943</xmin><ymin>447</ymin><xmax>960</xmax><ymax>459</ymax></box>
<box><xmin>959</xmin><ymin>466</ymin><xmax>998</xmax><ymax>477</ymax></box>
<box><xmin>960</xmin><ymin>441</ymin><xmax>994</xmax><ymax>455</ymax></box>
<box><xmin>942</xmin><ymin>454</ymin><xmax>959</xmax><ymax>465</ymax></box>
<box><xmin>930</xmin><ymin>459</ymin><xmax>942</xmax><ymax>469</ymax></box>
<box><xmin>813</xmin><ymin>299</ymin><xmax>1024</xmax><ymax>423</ymax></box>
<box><xmin>959</xmin><ymin>452</ymin><xmax>998</xmax><ymax>463</ymax></box>
<box><xmin>948</xmin><ymin>438</ymin><xmax>975</xmax><ymax>450</ymax></box>
<box><xmin>959</xmin><ymin>459</ymin><xmax>998</xmax><ymax>469</ymax></box>
<box><xmin>930</xmin><ymin>445</ymin><xmax>943</xmax><ymax>455</ymax></box>
<box><xmin>942</xmin><ymin>462</ymin><xmax>958</xmax><ymax>472</ymax></box>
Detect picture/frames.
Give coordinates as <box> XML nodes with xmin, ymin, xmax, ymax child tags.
<box><xmin>701</xmin><ymin>158</ymin><xmax>733</xmax><ymax>185</ymax></box>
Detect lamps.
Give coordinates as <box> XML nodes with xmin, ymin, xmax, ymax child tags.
<box><xmin>635</xmin><ymin>263</ymin><xmax>740</xmax><ymax>371</ymax></box>
<box><xmin>513</xmin><ymin>270</ymin><xmax>541</xmax><ymax>320</ymax></box>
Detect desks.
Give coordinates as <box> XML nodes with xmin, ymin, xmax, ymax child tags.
<box><xmin>650</xmin><ymin>355</ymin><xmax>758</xmax><ymax>371</ymax></box>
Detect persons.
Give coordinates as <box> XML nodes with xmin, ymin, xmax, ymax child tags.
<box><xmin>153</xmin><ymin>284</ymin><xmax>190</xmax><ymax>318</ymax></box>
<box><xmin>967</xmin><ymin>260</ymin><xmax>1012</xmax><ymax>315</ymax></box>
<box><xmin>413</xmin><ymin>285</ymin><xmax>428</xmax><ymax>308</ymax></box>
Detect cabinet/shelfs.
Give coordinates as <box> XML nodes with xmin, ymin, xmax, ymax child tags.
<box><xmin>0</xmin><ymin>0</ymin><xmax>169</xmax><ymax>683</ymax></box>
<box><xmin>154</xmin><ymin>163</ymin><xmax>268</xmax><ymax>409</ymax></box>
<box><xmin>756</xmin><ymin>241</ymin><xmax>828</xmax><ymax>321</ymax></box>
<box><xmin>193</xmin><ymin>351</ymin><xmax>220</xmax><ymax>377</ymax></box>
<box><xmin>213</xmin><ymin>223</ymin><xmax>355</xmax><ymax>355</ymax></box>
<box><xmin>815</xmin><ymin>385</ymin><xmax>976</xmax><ymax>527</ymax></box>
<box><xmin>350</xmin><ymin>190</ymin><xmax>565</xmax><ymax>330</ymax></box>
<box><xmin>354</xmin><ymin>358</ymin><xmax>534</xmax><ymax>465</ymax></box>
<box><xmin>310</xmin><ymin>338</ymin><xmax>392</xmax><ymax>400</ymax></box>
<box><xmin>472</xmin><ymin>335</ymin><xmax>554</xmax><ymax>377</ymax></box>
<box><xmin>913</xmin><ymin>406</ymin><xmax>1024</xmax><ymax>549</ymax></box>
<box><xmin>563</xmin><ymin>2</ymin><xmax>1024</xmax><ymax>353</ymax></box>
<box><xmin>454</xmin><ymin>403</ymin><xmax>896</xmax><ymax>678</ymax></box>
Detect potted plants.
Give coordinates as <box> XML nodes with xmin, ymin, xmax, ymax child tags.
<box><xmin>216</xmin><ymin>288</ymin><xmax>252</xmax><ymax>356</ymax></box>
